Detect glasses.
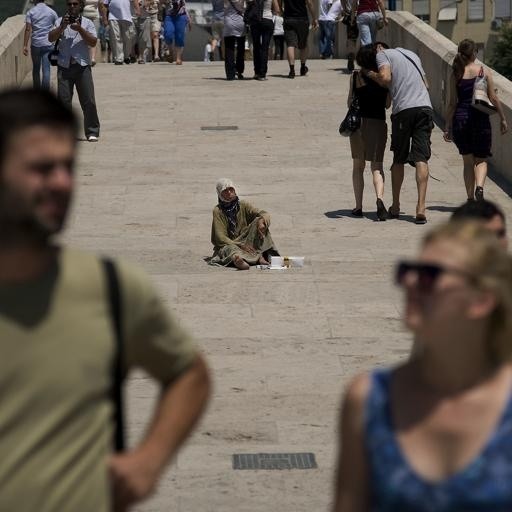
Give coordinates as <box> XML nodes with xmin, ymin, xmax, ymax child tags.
<box><xmin>394</xmin><ymin>261</ymin><xmax>473</xmax><ymax>290</ymax></box>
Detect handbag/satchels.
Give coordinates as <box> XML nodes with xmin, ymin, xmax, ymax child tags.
<box><xmin>472</xmin><ymin>76</ymin><xmax>498</xmax><ymax>114</ymax></box>
<box><xmin>48</xmin><ymin>49</ymin><xmax>59</xmax><ymax>66</ymax></box>
<box><xmin>339</xmin><ymin>100</ymin><xmax>360</xmax><ymax>137</ymax></box>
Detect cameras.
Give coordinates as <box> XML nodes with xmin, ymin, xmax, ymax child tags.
<box><xmin>68</xmin><ymin>12</ymin><xmax>78</xmax><ymax>25</ymax></box>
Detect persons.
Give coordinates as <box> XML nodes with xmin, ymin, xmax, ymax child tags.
<box><xmin>0</xmin><ymin>86</ymin><xmax>212</xmax><ymax>511</ymax></box>
<box><xmin>84</xmin><ymin>0</ymin><xmax>192</xmax><ymax>64</ymax></box>
<box><xmin>23</xmin><ymin>1</ymin><xmax>59</xmax><ymax>86</ymax></box>
<box><xmin>449</xmin><ymin>196</ymin><xmax>506</xmax><ymax>254</ymax></box>
<box><xmin>348</xmin><ymin>44</ymin><xmax>393</xmax><ymax>221</ymax></box>
<box><xmin>332</xmin><ymin>221</ymin><xmax>510</xmax><ymax>511</ymax></box>
<box><xmin>210</xmin><ymin>177</ymin><xmax>279</xmax><ymax>269</ymax></box>
<box><xmin>352</xmin><ymin>40</ymin><xmax>433</xmax><ymax>225</ymax></box>
<box><xmin>205</xmin><ymin>0</ymin><xmax>389</xmax><ymax>82</ymax></box>
<box><xmin>48</xmin><ymin>0</ymin><xmax>100</xmax><ymax>140</ymax></box>
<box><xmin>442</xmin><ymin>40</ymin><xmax>510</xmax><ymax>206</ymax></box>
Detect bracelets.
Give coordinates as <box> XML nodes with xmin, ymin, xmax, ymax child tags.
<box><xmin>442</xmin><ymin>127</ymin><xmax>451</xmax><ymax>132</ymax></box>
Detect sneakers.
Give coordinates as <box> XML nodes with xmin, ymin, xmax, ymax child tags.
<box><xmin>88</xmin><ymin>136</ymin><xmax>97</xmax><ymax>141</ymax></box>
<box><xmin>352</xmin><ymin>187</ymin><xmax>484</xmax><ymax>224</ymax></box>
